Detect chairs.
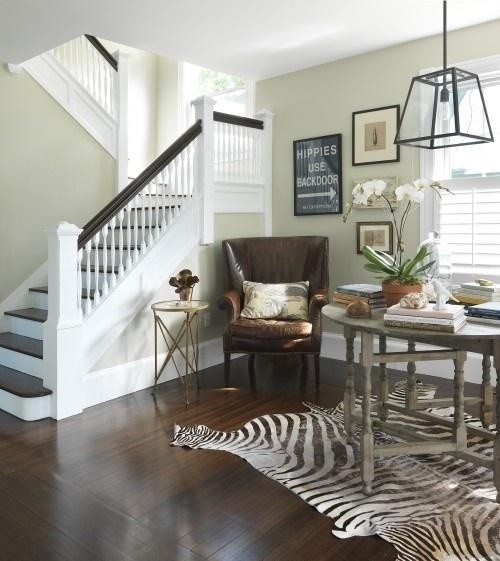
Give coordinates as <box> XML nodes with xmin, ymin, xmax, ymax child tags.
<box><xmin>218</xmin><ymin>235</ymin><xmax>329</xmax><ymax>389</ymax></box>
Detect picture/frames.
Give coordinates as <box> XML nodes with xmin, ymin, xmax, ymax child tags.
<box><xmin>292</xmin><ymin>133</ymin><xmax>342</xmax><ymax>216</ymax></box>
<box><xmin>356</xmin><ymin>221</ymin><xmax>393</xmax><ymax>255</ymax></box>
<box><xmin>348</xmin><ymin>105</ymin><xmax>400</xmax><ymax>166</ymax></box>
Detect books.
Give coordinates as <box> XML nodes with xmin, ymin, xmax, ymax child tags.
<box><xmin>383</xmin><ymin>302</ymin><xmax>468</xmax><ymax>334</ymax></box>
<box><xmin>449</xmin><ymin>282</ymin><xmax>500</xmax><ymax>305</ymax></box>
<box><xmin>464</xmin><ymin>302</ymin><xmax>500</xmax><ymax>325</ymax></box>
<box><xmin>332</xmin><ymin>284</ymin><xmax>387</xmax><ymax>309</ymax></box>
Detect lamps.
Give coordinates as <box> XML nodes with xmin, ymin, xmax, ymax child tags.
<box><xmin>394</xmin><ymin>2</ymin><xmax>492</xmax><ymax>151</ymax></box>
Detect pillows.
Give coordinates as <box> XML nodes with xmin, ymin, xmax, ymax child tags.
<box><xmin>241</xmin><ymin>280</ymin><xmax>309</xmax><ymax>319</ymax></box>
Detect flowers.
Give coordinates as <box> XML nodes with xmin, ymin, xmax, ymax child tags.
<box><xmin>345</xmin><ymin>179</ymin><xmax>433</xmax><ymax>280</ymax></box>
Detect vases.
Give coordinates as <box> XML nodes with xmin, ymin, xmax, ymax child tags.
<box><xmin>384</xmin><ymin>283</ymin><xmax>422</xmax><ymax>308</ymax></box>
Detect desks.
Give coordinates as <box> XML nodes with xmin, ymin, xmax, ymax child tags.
<box><xmin>151</xmin><ymin>300</ymin><xmax>208</xmax><ymax>408</ymax></box>
<box><xmin>322</xmin><ymin>300</ymin><xmax>500</xmax><ymax>501</ymax></box>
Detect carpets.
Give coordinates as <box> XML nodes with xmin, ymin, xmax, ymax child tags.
<box><xmin>169</xmin><ymin>381</ymin><xmax>498</xmax><ymax>561</ymax></box>
